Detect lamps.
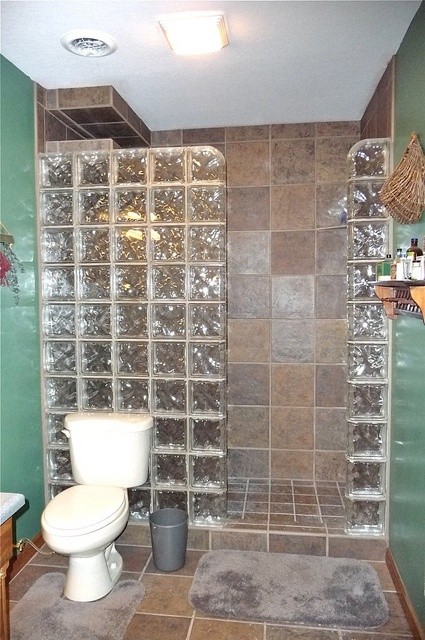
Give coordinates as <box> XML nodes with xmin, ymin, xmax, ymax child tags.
<box><xmin>159</xmin><ymin>12</ymin><xmax>230</xmax><ymax>55</ymax></box>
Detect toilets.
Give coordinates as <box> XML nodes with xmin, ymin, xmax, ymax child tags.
<box><xmin>40</xmin><ymin>411</ymin><xmax>154</xmax><ymax>602</ymax></box>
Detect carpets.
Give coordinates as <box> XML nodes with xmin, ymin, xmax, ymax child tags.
<box><xmin>189</xmin><ymin>550</ymin><xmax>389</xmax><ymax>629</ymax></box>
<box><xmin>9</xmin><ymin>572</ymin><xmax>144</xmax><ymax>639</ymax></box>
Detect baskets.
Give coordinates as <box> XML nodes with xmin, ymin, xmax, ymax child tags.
<box><xmin>378</xmin><ymin>131</ymin><xmax>425</xmax><ymax>224</ymax></box>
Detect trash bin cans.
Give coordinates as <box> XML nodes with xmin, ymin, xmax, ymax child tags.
<box><xmin>147</xmin><ymin>508</ymin><xmax>188</xmax><ymax>571</ymax></box>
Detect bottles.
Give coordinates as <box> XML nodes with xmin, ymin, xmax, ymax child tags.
<box><xmin>406</xmin><ymin>238</ymin><xmax>423</xmax><ymax>280</ymax></box>
<box><xmin>381</xmin><ymin>254</ymin><xmax>393</xmax><ymax>275</ymax></box>
<box><xmin>411</xmin><ymin>260</ymin><xmax>422</xmax><ymax>279</ymax></box>
<box><xmin>391</xmin><ymin>249</ymin><xmax>407</xmax><ymax>279</ymax></box>
<box><xmin>416</xmin><ymin>253</ymin><xmax>425</xmax><ymax>279</ymax></box>
